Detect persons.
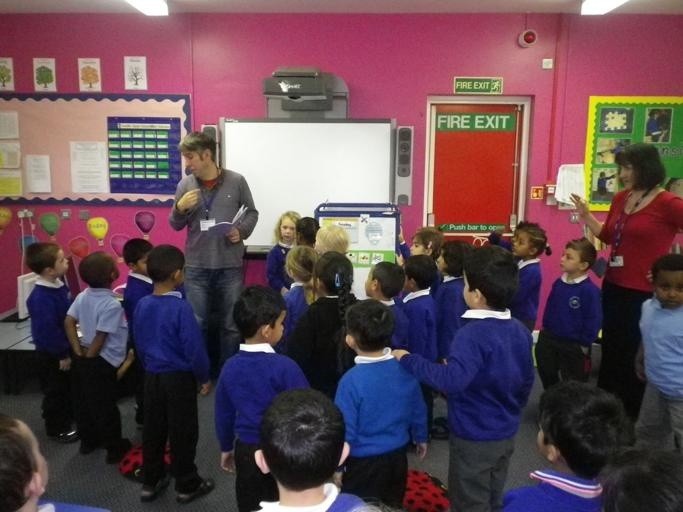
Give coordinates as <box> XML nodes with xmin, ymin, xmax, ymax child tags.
<box><xmin>596</xmin><ymin>168</ymin><xmax>617</xmax><ymax>197</ymax></box>
<box><xmin>570</xmin><ymin>141</ymin><xmax>683</xmax><ymax>425</ymax></box>
<box><xmin>168</xmin><ymin>131</ymin><xmax>259</xmax><ymax>397</ymax></box>
<box><xmin>0</xmin><ymin>209</ymin><xmax>682</xmax><ymax>512</ymax></box>
<box><xmin>365</xmin><ymin>220</ymin><xmax>387</xmax><ymax>247</ymax></box>
<box><xmin>649</xmin><ymin>113</ymin><xmax>665</xmax><ymax>138</ymax></box>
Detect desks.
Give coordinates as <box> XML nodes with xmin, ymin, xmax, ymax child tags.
<box><xmin>0</xmin><ymin>307</ymin><xmax>33</xmax><ymax>395</ymax></box>
<box><xmin>8</xmin><ymin>335</ymin><xmax>37</xmax><ymax>395</ymax></box>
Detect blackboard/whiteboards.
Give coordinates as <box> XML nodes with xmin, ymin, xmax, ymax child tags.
<box><xmin>219</xmin><ymin>118</ymin><xmax>397</xmax><ymax>259</ymax></box>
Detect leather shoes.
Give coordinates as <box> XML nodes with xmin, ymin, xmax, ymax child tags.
<box><xmin>45</xmin><ymin>428</ymin><xmax>78</xmax><ymax>442</ymax></box>
<box><xmin>432</xmin><ymin>426</ymin><xmax>450</xmax><ymax>439</ymax></box>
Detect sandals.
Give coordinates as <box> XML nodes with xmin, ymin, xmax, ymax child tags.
<box><xmin>177</xmin><ymin>478</ymin><xmax>214</xmax><ymax>503</ymax></box>
<box><xmin>141</xmin><ymin>477</ymin><xmax>169</xmax><ymax>500</ymax></box>
